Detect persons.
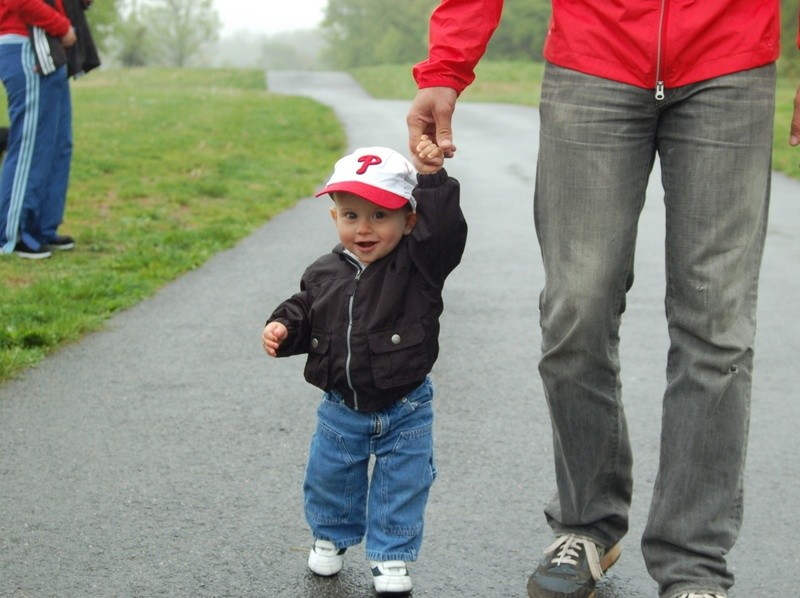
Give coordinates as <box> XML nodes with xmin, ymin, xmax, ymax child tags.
<box><xmin>0</xmin><ymin>0</ymin><xmax>78</xmax><ymax>259</ymax></box>
<box><xmin>405</xmin><ymin>0</ymin><xmax>800</xmax><ymax>598</ymax></box>
<box><xmin>262</xmin><ymin>135</ymin><xmax>469</xmax><ymax>592</ymax></box>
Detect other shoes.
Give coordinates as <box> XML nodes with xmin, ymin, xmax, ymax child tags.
<box><xmin>13</xmin><ymin>241</ymin><xmax>52</xmax><ymax>258</ymax></box>
<box><xmin>46</xmin><ymin>236</ymin><xmax>74</xmax><ymax>249</ymax></box>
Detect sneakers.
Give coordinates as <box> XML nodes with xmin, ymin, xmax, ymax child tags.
<box><xmin>370</xmin><ymin>560</ymin><xmax>412</xmax><ymax>593</ymax></box>
<box><xmin>308</xmin><ymin>539</ymin><xmax>347</xmax><ymax>576</ymax></box>
<box><xmin>670</xmin><ymin>593</ymin><xmax>727</xmax><ymax>598</ymax></box>
<box><xmin>526</xmin><ymin>536</ymin><xmax>622</xmax><ymax>598</ymax></box>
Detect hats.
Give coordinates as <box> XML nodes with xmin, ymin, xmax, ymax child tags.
<box><xmin>316</xmin><ymin>146</ymin><xmax>418</xmax><ymax>213</ymax></box>
